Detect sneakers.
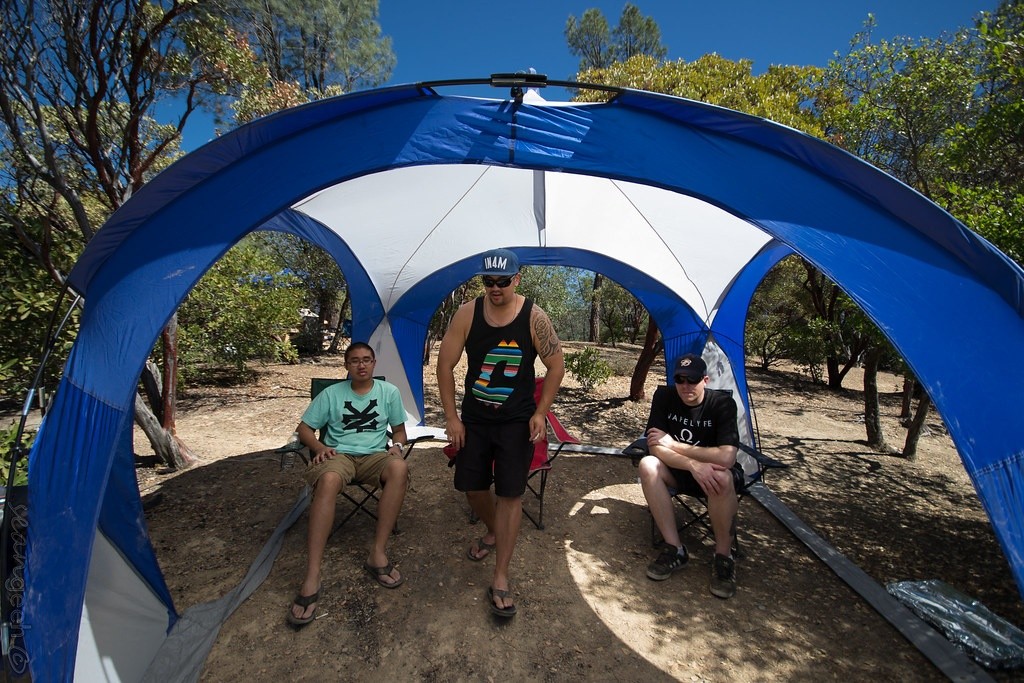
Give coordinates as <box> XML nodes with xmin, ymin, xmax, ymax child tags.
<box><xmin>710</xmin><ymin>553</ymin><xmax>737</xmax><ymax>598</ymax></box>
<box><xmin>646</xmin><ymin>542</ymin><xmax>689</xmax><ymax>581</ymax></box>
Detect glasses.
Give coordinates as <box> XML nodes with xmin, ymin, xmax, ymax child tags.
<box><xmin>675</xmin><ymin>375</ymin><xmax>696</xmax><ymax>384</ymax></box>
<box><xmin>483</xmin><ymin>275</ymin><xmax>517</xmax><ymax>288</ymax></box>
<box><xmin>346</xmin><ymin>359</ymin><xmax>373</xmax><ymax>366</ymax></box>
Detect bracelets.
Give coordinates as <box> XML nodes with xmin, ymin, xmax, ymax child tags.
<box><xmin>394</xmin><ymin>442</ymin><xmax>404</xmax><ymax>454</ymax></box>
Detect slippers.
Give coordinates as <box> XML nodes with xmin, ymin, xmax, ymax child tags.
<box><xmin>362</xmin><ymin>556</ymin><xmax>403</xmax><ymax>588</ymax></box>
<box><xmin>484</xmin><ymin>586</ymin><xmax>517</xmax><ymax>617</ymax></box>
<box><xmin>467</xmin><ymin>537</ymin><xmax>496</xmax><ymax>561</ymax></box>
<box><xmin>287</xmin><ymin>583</ymin><xmax>324</xmax><ymax>625</ymax></box>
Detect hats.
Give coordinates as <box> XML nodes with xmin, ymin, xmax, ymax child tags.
<box><xmin>476</xmin><ymin>248</ymin><xmax>519</xmax><ymax>275</ymax></box>
<box><xmin>675</xmin><ymin>353</ymin><xmax>707</xmax><ymax>375</ymax></box>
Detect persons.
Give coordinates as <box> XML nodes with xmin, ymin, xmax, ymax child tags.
<box><xmin>436</xmin><ymin>249</ymin><xmax>564</xmax><ymax>617</ymax></box>
<box><xmin>637</xmin><ymin>354</ymin><xmax>746</xmax><ymax>599</ymax></box>
<box><xmin>288</xmin><ymin>342</ymin><xmax>406</xmax><ymax>626</ymax></box>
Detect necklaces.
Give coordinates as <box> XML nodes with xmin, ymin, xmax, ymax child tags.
<box><xmin>484</xmin><ymin>293</ymin><xmax>517</xmax><ymax>328</ymax></box>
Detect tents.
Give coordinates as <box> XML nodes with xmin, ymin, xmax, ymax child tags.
<box><xmin>0</xmin><ymin>63</ymin><xmax>1024</xmax><ymax>683</ymax></box>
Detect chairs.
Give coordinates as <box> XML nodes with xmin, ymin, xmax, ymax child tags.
<box><xmin>443</xmin><ymin>377</ymin><xmax>580</xmax><ymax>530</ymax></box>
<box><xmin>273</xmin><ymin>375</ymin><xmax>435</xmax><ymax>541</ymax></box>
<box><xmin>621</xmin><ymin>386</ymin><xmax>790</xmax><ymax>558</ymax></box>
